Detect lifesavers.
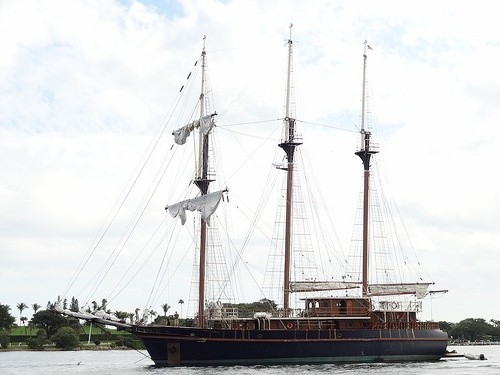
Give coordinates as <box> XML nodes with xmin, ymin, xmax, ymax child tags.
<box><xmin>286</xmin><ymin>322</ymin><xmax>293</xmax><ymax>329</ymax></box>
<box><xmin>391</xmin><ymin>301</ymin><xmax>397</xmax><ymax>309</ymax></box>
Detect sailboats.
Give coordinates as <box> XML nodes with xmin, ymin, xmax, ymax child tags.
<box><xmin>51</xmin><ymin>24</ymin><xmax>450</xmax><ymax>365</ymax></box>
